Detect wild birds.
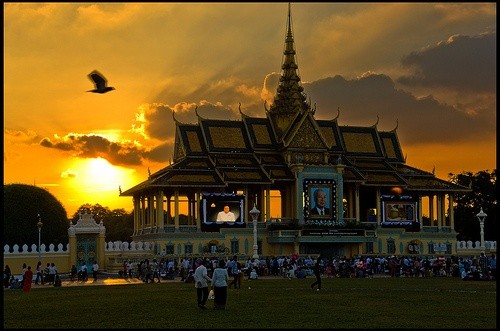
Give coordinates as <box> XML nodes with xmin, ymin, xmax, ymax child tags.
<box><xmin>83</xmin><ymin>70</ymin><xmax>116</xmax><ymax>95</ymax></box>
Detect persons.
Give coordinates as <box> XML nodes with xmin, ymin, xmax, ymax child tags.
<box><xmin>216</xmin><ymin>204</ymin><xmax>236</xmax><ymax>222</ymax></box>
<box><xmin>211</xmin><ymin>258</ymin><xmax>231</xmax><ymax>311</ymax></box>
<box><xmin>312</xmin><ymin>189</ymin><xmax>330</xmax><ymax>215</ymax></box>
<box><xmin>3</xmin><ymin>248</ymin><xmax>497</xmax><ymax>292</ymax></box>
<box><xmin>193</xmin><ymin>261</ymin><xmax>211</xmax><ymax>309</ymax></box>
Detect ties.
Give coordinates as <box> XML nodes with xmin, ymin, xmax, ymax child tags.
<box><xmin>321</xmin><ymin>210</ymin><xmax>324</xmax><ymax>215</ymax></box>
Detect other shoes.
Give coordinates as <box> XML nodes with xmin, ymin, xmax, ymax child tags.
<box><xmin>311</xmin><ymin>285</ymin><xmax>314</xmax><ymax>288</ymax></box>
<box><xmin>181</xmin><ymin>280</ymin><xmax>184</xmax><ymax>281</ymax></box>
